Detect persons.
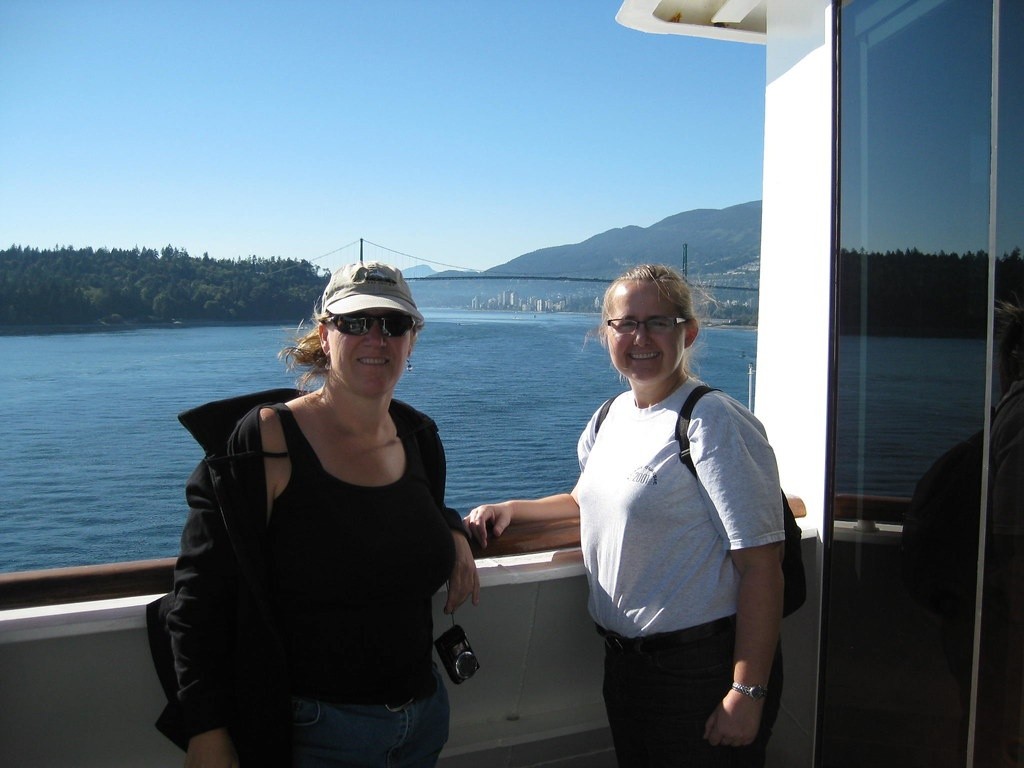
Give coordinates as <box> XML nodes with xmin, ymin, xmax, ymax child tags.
<box><xmin>162</xmin><ymin>257</ymin><xmax>481</xmax><ymax>768</ymax></box>
<box><xmin>462</xmin><ymin>262</ymin><xmax>786</xmax><ymax>768</ymax></box>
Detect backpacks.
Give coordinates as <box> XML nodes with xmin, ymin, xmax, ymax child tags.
<box><xmin>898</xmin><ymin>387</ymin><xmax>1024</xmax><ymax>614</ymax></box>
<box><xmin>596</xmin><ymin>386</ymin><xmax>807</xmax><ymax>619</ymax></box>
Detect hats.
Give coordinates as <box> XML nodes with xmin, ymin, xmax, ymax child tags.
<box><xmin>322</xmin><ymin>261</ymin><xmax>424</xmax><ymax>328</ymax></box>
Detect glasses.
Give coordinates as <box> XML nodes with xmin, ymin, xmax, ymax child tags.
<box><xmin>332</xmin><ymin>313</ymin><xmax>415</xmax><ymax>339</ymax></box>
<box><xmin>606</xmin><ymin>317</ymin><xmax>686</xmax><ymax>336</ymax></box>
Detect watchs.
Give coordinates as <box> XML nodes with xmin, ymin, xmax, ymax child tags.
<box><xmin>729</xmin><ymin>682</ymin><xmax>768</xmax><ymax>700</ymax></box>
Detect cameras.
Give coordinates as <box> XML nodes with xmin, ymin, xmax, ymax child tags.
<box><xmin>434</xmin><ymin>624</ymin><xmax>480</xmax><ymax>685</ymax></box>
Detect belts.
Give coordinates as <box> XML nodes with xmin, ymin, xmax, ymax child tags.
<box><xmin>603</xmin><ymin>617</ymin><xmax>736</xmax><ymax>658</ymax></box>
